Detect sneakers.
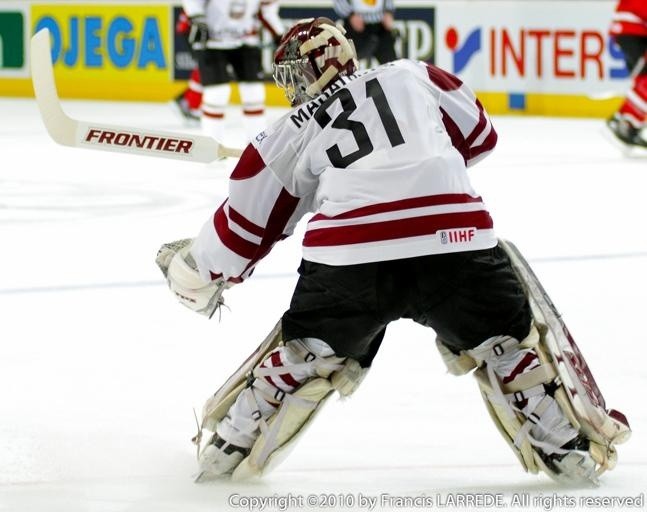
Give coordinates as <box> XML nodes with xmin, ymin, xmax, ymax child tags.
<box><xmin>608</xmin><ymin>112</ymin><xmax>646</xmax><ymax>147</ymax></box>
<box><xmin>549</xmin><ymin>437</ymin><xmax>594</xmax><ymax>480</ymax></box>
<box><xmin>201</xmin><ymin>433</ymin><xmax>246</xmax><ymax>474</ymax></box>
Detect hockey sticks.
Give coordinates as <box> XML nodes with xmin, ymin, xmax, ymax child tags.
<box><xmin>30</xmin><ymin>27</ymin><xmax>246</xmax><ymax>165</ymax></box>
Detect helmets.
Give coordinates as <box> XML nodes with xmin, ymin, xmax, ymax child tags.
<box><xmin>272</xmin><ymin>17</ymin><xmax>358</xmax><ymax>105</ymax></box>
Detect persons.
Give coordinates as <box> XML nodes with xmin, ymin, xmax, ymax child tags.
<box><xmin>606</xmin><ymin>1</ymin><xmax>647</xmax><ymax>147</ymax></box>
<box><xmin>173</xmin><ymin>0</ymin><xmax>398</xmax><ymax>120</ymax></box>
<box><xmin>155</xmin><ymin>15</ymin><xmax>631</xmax><ymax>483</ymax></box>
